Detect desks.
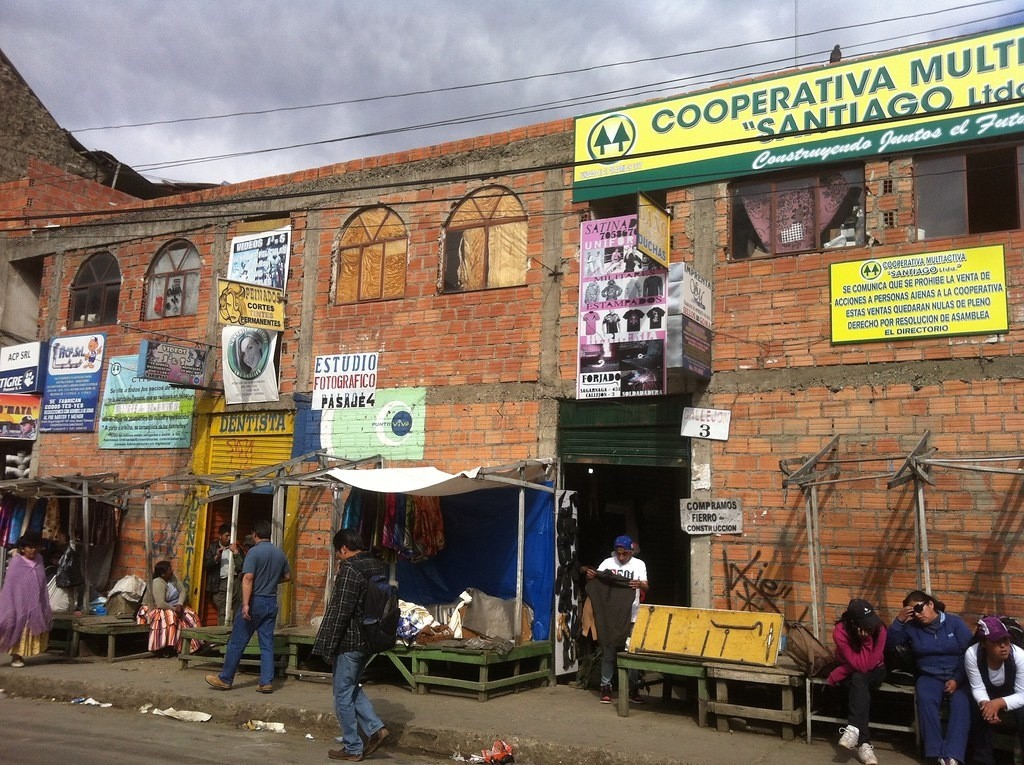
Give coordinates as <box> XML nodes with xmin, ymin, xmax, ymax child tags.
<box><xmin>616</xmin><ymin>652</ymin><xmax>922</xmax><ymax>749</ymax></box>
<box><xmin>177</xmin><ymin>625</ymin><xmax>552</xmax><ymax>704</ymax></box>
<box><xmin>47</xmin><ymin>612</ymin><xmax>153</xmax><ymax>663</ymax></box>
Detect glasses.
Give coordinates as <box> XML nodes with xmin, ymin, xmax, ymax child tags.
<box><xmin>909</xmin><ymin>601</ymin><xmax>927</xmax><ymax>617</ymax></box>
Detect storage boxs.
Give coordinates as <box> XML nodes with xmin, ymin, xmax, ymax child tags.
<box><xmin>829</xmin><ymin>230</ymin><xmax>856</xmax><ymax>248</ymax></box>
<box><xmin>73</xmin><ymin>320</ymin><xmax>93</xmax><ymax>327</ymax></box>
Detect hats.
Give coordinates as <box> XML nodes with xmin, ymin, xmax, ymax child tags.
<box><xmin>614</xmin><ymin>535</ymin><xmax>634</xmax><ymax>552</ymax></box>
<box><xmin>847</xmin><ymin>599</ymin><xmax>882</xmax><ymax>629</ymax></box>
<box><xmin>977</xmin><ymin>616</ymin><xmax>1012</xmax><ymax>640</ymax></box>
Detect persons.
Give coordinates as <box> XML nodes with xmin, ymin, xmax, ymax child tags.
<box><xmin>585</xmin><ymin>245</ymin><xmax>643</xmax><ymax>275</ymax></box>
<box><xmin>601</xmin><ymin>309</ymin><xmax>622</xmax><ymax>335</ymax></box>
<box><xmin>204</xmin><ymin>520</ymin><xmax>293</xmax><ymax>694</ymax></box>
<box><xmin>584</xmin><ymin>535</ymin><xmax>650</xmax><ymax>704</ymax></box>
<box><xmin>207</xmin><ymin>525</ymin><xmax>244</xmax><ymax>627</ymax></box>
<box><xmin>962</xmin><ymin>615</ymin><xmax>1024</xmax><ymax>765</ymax></box>
<box><xmin>826</xmin><ymin>598</ymin><xmax>889</xmax><ymax>765</ymax></box>
<box><xmin>888</xmin><ymin>590</ymin><xmax>975</xmax><ymax>765</ymax></box>
<box><xmin>312</xmin><ymin>528</ymin><xmax>389</xmax><ymax>762</ymax></box>
<box><xmin>234</xmin><ymin>331</ymin><xmax>264</xmax><ymax>375</ymax></box>
<box><xmin>136</xmin><ymin>560</ymin><xmax>203</xmax><ymax>657</ymax></box>
<box><xmin>20</xmin><ymin>416</ymin><xmax>36</xmax><ymax>441</ymax></box>
<box><xmin>0</xmin><ymin>534</ymin><xmax>52</xmax><ymax>668</ymax></box>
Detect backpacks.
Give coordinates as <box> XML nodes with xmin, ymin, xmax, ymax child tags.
<box><xmin>341</xmin><ymin>560</ymin><xmax>401</xmax><ymax>654</ymax></box>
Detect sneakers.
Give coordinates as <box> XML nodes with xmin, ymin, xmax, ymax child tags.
<box><xmin>205</xmin><ymin>675</ymin><xmax>231</xmax><ymax>690</ymax></box>
<box><xmin>838</xmin><ymin>725</ymin><xmax>859</xmax><ymax>751</ymax></box>
<box><xmin>858</xmin><ymin>743</ymin><xmax>878</xmax><ymax>765</ymax></box>
<box><xmin>364</xmin><ymin>727</ymin><xmax>392</xmax><ymax>757</ymax></box>
<box><xmin>328</xmin><ymin>747</ymin><xmax>363</xmax><ymax>762</ymax></box>
<box><xmin>255</xmin><ymin>683</ymin><xmax>273</xmax><ymax>693</ymax></box>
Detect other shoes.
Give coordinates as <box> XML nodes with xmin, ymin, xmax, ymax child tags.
<box><xmin>600</xmin><ymin>686</ymin><xmax>612</xmax><ymax>704</ymax></box>
<box><xmin>10</xmin><ymin>661</ymin><xmax>25</xmax><ymax>668</ymax></box>
<box><xmin>938</xmin><ymin>757</ymin><xmax>958</xmax><ymax>765</ymax></box>
<box><xmin>629</xmin><ymin>695</ymin><xmax>644</xmax><ymax>704</ymax></box>
